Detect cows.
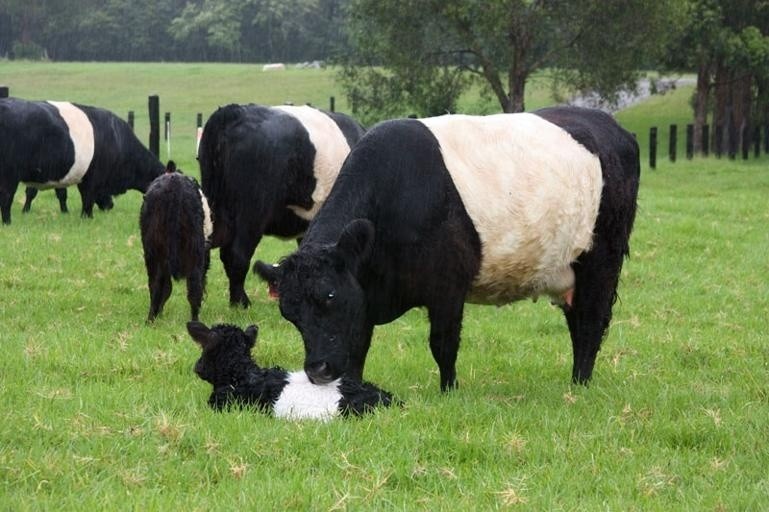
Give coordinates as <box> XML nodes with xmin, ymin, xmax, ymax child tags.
<box><xmin>138</xmin><ymin>157</ymin><xmax>215</xmax><ymax>330</ymax></box>
<box><xmin>0</xmin><ymin>95</ymin><xmax>184</xmax><ymax>225</ymax></box>
<box><xmin>194</xmin><ymin>101</ymin><xmax>418</xmax><ymax>310</ymax></box>
<box><xmin>182</xmin><ymin>319</ymin><xmax>404</xmax><ymax>419</ymax></box>
<box><xmin>252</xmin><ymin>101</ymin><xmax>642</xmax><ymax>398</ymax></box>
